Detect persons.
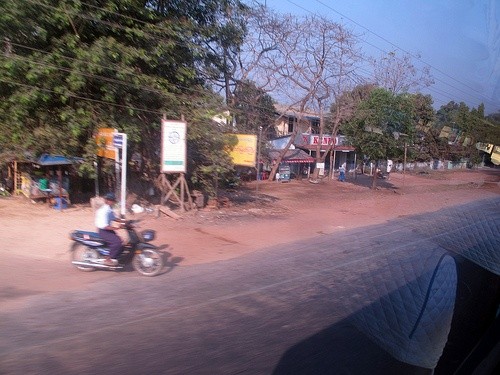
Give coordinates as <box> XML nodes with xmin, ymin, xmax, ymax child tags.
<box><xmin>337</xmin><ymin>166</ymin><xmax>346</xmax><ymax>182</ymax></box>
<box><xmin>39</xmin><ymin>175</ymin><xmax>51</xmax><ymax>208</ymax></box>
<box><xmin>95</xmin><ymin>192</ymin><xmax>130</xmax><ymax>265</ymax></box>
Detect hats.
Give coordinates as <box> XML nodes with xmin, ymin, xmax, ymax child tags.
<box><xmin>103</xmin><ymin>191</ymin><xmax>118</xmax><ymax>203</ymax></box>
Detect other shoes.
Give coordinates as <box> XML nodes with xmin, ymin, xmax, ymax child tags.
<box><xmin>102</xmin><ymin>256</ymin><xmax>116</xmax><ymax>266</ymax></box>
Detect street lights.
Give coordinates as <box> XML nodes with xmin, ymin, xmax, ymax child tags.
<box><xmin>392</xmin><ymin>130</ymin><xmax>409</xmax><ymax>188</ymax></box>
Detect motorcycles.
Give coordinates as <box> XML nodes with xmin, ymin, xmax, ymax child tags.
<box><xmin>64</xmin><ymin>215</ymin><xmax>164</xmax><ymax>276</ymax></box>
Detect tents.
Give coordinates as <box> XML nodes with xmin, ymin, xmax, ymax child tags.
<box><xmin>262</xmin><ymin>148</ymin><xmax>314</xmax><ymax>180</ymax></box>
